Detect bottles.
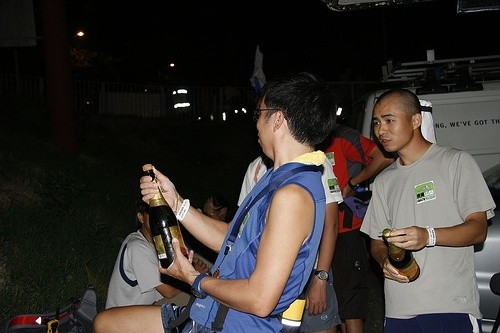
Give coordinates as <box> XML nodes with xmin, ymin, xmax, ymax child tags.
<box><xmin>382</xmin><ymin>228</ymin><xmax>420</xmax><ymax>282</ymax></box>
<box><xmin>143</xmin><ymin>163</ymin><xmax>189</xmax><ymax>269</ymax></box>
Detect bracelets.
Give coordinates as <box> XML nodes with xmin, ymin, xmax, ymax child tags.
<box><xmin>426</xmin><ymin>226</ymin><xmax>436</xmax><ymax>247</ymax></box>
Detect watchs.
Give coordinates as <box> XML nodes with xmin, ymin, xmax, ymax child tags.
<box><xmin>191</xmin><ymin>272</ymin><xmax>208</xmax><ymax>299</ymax></box>
<box><xmin>348</xmin><ymin>178</ymin><xmax>360</xmax><ymax>191</ymax></box>
<box><xmin>314</xmin><ymin>270</ymin><xmax>330</xmax><ymax>282</ymax></box>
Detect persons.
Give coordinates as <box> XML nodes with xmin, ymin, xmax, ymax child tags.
<box><xmin>325</xmin><ymin>123</ymin><xmax>396</xmax><ymax>332</ymax></box>
<box><xmin>105</xmin><ymin>197</ymin><xmax>194</xmax><ymax>311</ymax></box>
<box><xmin>358</xmin><ymin>89</ymin><xmax>496</xmax><ymax>333</ymax></box>
<box><xmin>236</xmin><ymin>83</ymin><xmax>344</xmax><ymax>332</ymax></box>
<box><xmin>92</xmin><ymin>72</ymin><xmax>336</xmax><ymax>332</ymax></box>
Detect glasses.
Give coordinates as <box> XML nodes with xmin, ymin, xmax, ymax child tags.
<box><xmin>255</xmin><ymin>107</ymin><xmax>291</xmax><ymax>123</ymax></box>
